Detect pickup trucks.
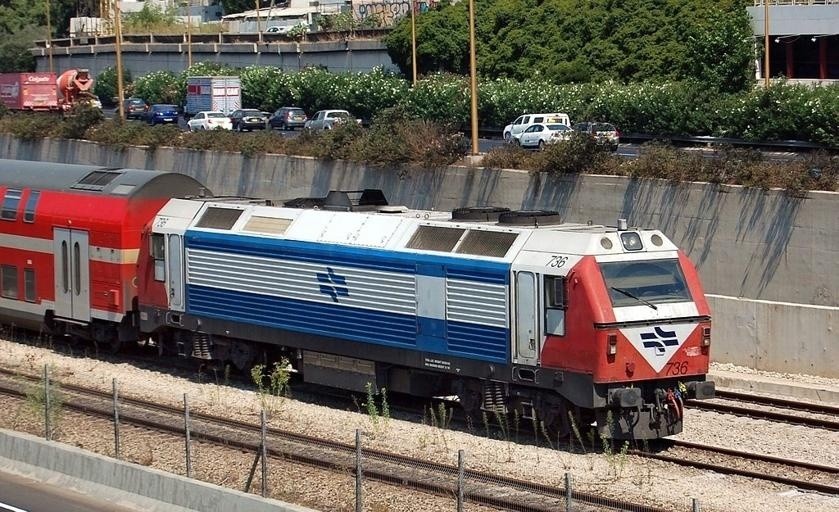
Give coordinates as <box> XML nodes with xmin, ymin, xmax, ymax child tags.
<box><xmin>304</xmin><ymin>109</ymin><xmax>363</xmax><ymax>131</ymax></box>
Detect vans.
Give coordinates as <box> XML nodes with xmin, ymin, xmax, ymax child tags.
<box><xmin>502</xmin><ymin>113</ymin><xmax>571</xmax><ymax>146</ymax></box>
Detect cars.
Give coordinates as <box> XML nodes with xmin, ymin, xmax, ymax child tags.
<box><xmin>186</xmin><ymin>107</ymin><xmax>308</xmax><ymax>132</ymax></box>
<box><xmin>510</xmin><ymin>123</ymin><xmax>575</xmax><ymax>151</ymax></box>
<box><xmin>266</xmin><ymin>26</ymin><xmax>284</xmax><ymax>32</ymax></box>
<box><xmin>571</xmin><ymin>122</ymin><xmax>620</xmax><ymax>154</ymax></box>
<box><xmin>115</xmin><ymin>97</ymin><xmax>182</xmax><ymax>126</ymax></box>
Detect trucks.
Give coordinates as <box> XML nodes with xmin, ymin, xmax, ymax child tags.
<box><xmin>180</xmin><ymin>76</ymin><xmax>241</xmax><ymax>120</ymax></box>
<box><xmin>1</xmin><ymin>73</ymin><xmax>58</xmax><ymax>111</ymax></box>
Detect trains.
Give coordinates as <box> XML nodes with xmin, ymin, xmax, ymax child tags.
<box><xmin>0</xmin><ymin>158</ymin><xmax>716</xmax><ymax>443</ymax></box>
